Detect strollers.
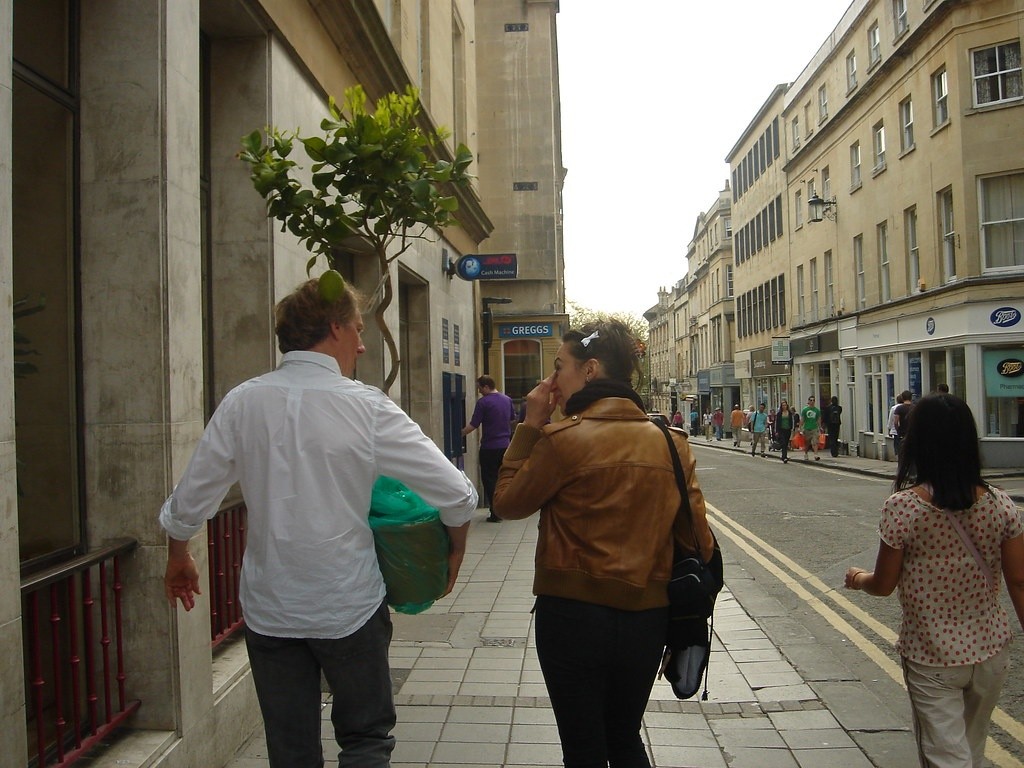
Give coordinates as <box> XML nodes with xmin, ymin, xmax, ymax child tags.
<box><xmin>768</xmin><ymin>422</ymin><xmax>782</xmax><ymax>451</ymax></box>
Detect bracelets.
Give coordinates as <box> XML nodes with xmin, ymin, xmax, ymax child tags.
<box><xmin>851</xmin><ymin>571</ymin><xmax>863</xmax><ymax>590</ymax></box>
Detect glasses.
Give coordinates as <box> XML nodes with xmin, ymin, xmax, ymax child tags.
<box><xmin>810</xmin><ymin>399</ymin><xmax>815</xmax><ymax>401</ymax></box>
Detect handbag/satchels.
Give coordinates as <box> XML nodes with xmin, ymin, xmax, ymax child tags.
<box><xmin>747</xmin><ymin>421</ymin><xmax>755</xmax><ymax>431</ymax></box>
<box><xmin>818</xmin><ymin>431</ymin><xmax>826</xmax><ymax>449</ymax></box>
<box><xmin>790</xmin><ymin>430</ymin><xmax>806</xmax><ymax>450</ymax></box>
<box><xmin>664</xmin><ymin>551</ymin><xmax>710</xmax><ymax>650</ymax></box>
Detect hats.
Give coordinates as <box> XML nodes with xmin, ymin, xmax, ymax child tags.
<box><xmin>715</xmin><ymin>407</ymin><xmax>721</xmax><ymax>411</ymax></box>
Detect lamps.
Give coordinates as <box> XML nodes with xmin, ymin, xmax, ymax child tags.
<box><xmin>807</xmin><ymin>189</ymin><xmax>837</xmax><ymax>223</ymax></box>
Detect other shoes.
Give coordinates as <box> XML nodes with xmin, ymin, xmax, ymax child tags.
<box><xmin>815</xmin><ymin>455</ymin><xmax>820</xmax><ymax>460</ymax></box>
<box><xmin>752</xmin><ymin>451</ymin><xmax>755</xmax><ymax>457</ymax></box>
<box><xmin>783</xmin><ymin>459</ymin><xmax>788</xmax><ymax>463</ymax></box>
<box><xmin>803</xmin><ymin>453</ymin><xmax>809</xmax><ymax>460</ymax></box>
<box><xmin>761</xmin><ymin>454</ymin><xmax>767</xmax><ymax>458</ymax></box>
<box><xmin>707</xmin><ymin>438</ymin><xmax>753</xmax><ymax>447</ymax></box>
<box><xmin>486</xmin><ymin>514</ymin><xmax>502</xmax><ymax>523</ymax></box>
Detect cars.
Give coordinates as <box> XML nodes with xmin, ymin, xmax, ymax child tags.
<box><xmin>647</xmin><ymin>412</ymin><xmax>670</xmax><ymax>426</ymax></box>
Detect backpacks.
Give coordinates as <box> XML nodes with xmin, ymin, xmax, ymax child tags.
<box><xmin>831</xmin><ymin>405</ymin><xmax>840</xmax><ymax>425</ymax></box>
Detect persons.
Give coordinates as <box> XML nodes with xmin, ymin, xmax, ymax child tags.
<box><xmin>460</xmin><ymin>374</ymin><xmax>517</xmax><ymax>523</ymax></box>
<box><xmin>887</xmin><ymin>383</ymin><xmax>949</xmax><ymax>483</ymax></box>
<box><xmin>157</xmin><ymin>279</ymin><xmax>478</xmax><ymax>768</ymax></box>
<box><xmin>672</xmin><ymin>407</ymin><xmax>725</xmax><ymax>441</ymax></box>
<box><xmin>845</xmin><ymin>393</ymin><xmax>1024</xmax><ymax>768</ymax></box>
<box><xmin>729</xmin><ymin>393</ymin><xmax>843</xmax><ymax>464</ymax></box>
<box><xmin>492</xmin><ymin>312</ymin><xmax>715</xmax><ymax>768</ymax></box>
